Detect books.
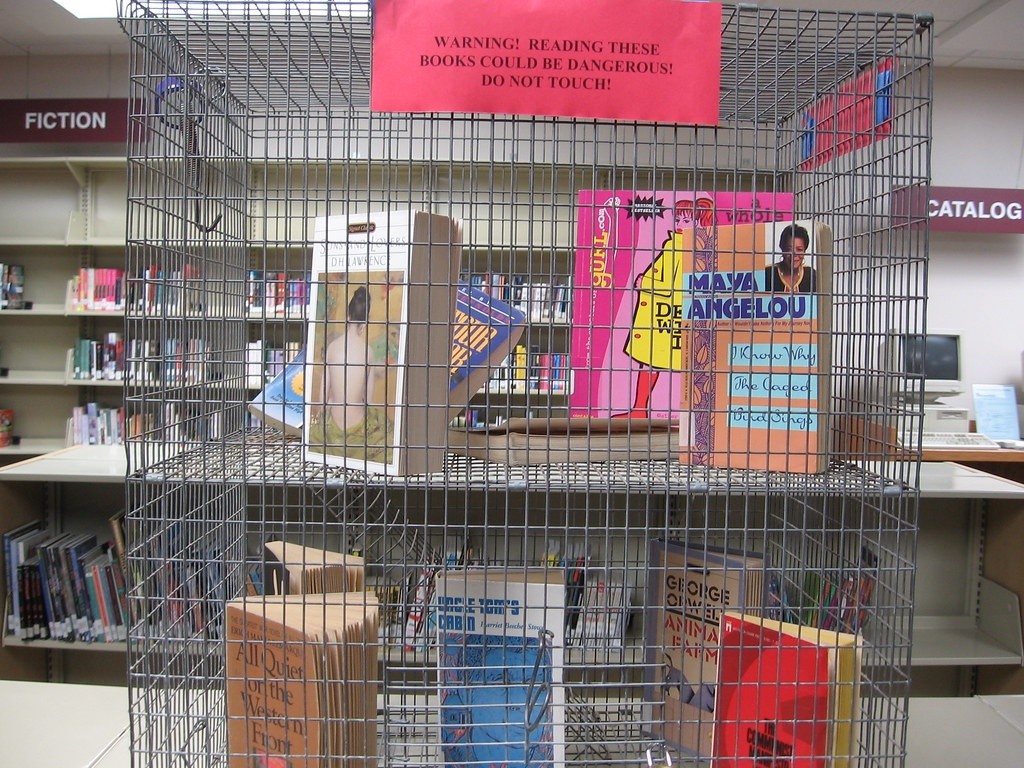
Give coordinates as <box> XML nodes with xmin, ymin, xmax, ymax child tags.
<box><xmin>0</xmin><ymin>188</ymin><xmax>838</xmax><ymax>475</ymax></box>
<box><xmin>0</xmin><ymin>509</ymin><xmax>882</xmax><ymax>768</ymax></box>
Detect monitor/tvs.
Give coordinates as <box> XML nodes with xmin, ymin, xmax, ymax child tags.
<box><xmin>878</xmin><ymin>327</ymin><xmax>962</xmax><ymax>407</ymax></box>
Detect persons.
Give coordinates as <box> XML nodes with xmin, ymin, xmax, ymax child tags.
<box><xmin>765</xmin><ymin>224</ymin><xmax>817</xmax><ymax>293</ymax></box>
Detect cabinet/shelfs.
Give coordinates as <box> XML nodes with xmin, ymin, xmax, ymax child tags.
<box><xmin>0</xmin><ymin>154</ymin><xmax>574</xmax><ymax>459</ymax></box>
<box><xmin>0</xmin><ymin>438</ymin><xmax>1023</xmax><ymax>768</ymax></box>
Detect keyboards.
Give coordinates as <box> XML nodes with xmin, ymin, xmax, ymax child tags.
<box><xmin>895</xmin><ymin>431</ymin><xmax>1001</xmax><ymax>451</ymax></box>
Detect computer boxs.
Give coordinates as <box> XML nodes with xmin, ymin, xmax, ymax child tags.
<box><xmin>871</xmin><ymin>401</ymin><xmax>979</xmax><ymax>434</ymax></box>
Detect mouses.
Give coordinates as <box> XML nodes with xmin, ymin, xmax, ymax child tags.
<box><xmin>998</xmin><ymin>441</ymin><xmax>1015</xmax><ymax>448</ymax></box>
